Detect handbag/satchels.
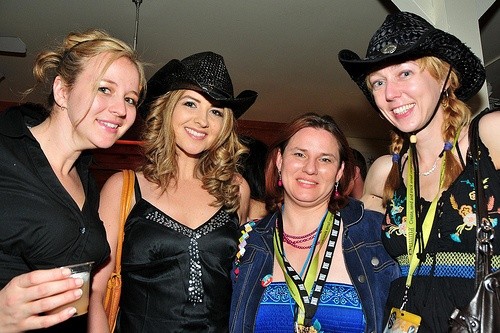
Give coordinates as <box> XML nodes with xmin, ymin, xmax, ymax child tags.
<box><xmin>449</xmin><ymin>268</ymin><xmax>500</xmax><ymax>333</ymax></box>
<box><xmin>101</xmin><ymin>169</ymin><xmax>136</xmax><ymax>333</ymax></box>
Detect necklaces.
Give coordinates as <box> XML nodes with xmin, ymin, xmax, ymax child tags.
<box><xmin>419</xmin><ymin>149</ymin><xmax>445</xmax><ymax>176</ymax></box>
<box><xmin>283</xmin><ymin>227</ymin><xmax>321</xmax><ymax>249</ymax></box>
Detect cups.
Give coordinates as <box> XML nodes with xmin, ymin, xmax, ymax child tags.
<box><xmin>44</xmin><ymin>261</ymin><xmax>95</xmax><ymax>317</ymax></box>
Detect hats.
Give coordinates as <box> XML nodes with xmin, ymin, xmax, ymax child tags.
<box><xmin>136</xmin><ymin>51</ymin><xmax>258</xmax><ymax>120</ymax></box>
<box><xmin>339</xmin><ymin>11</ymin><xmax>486</xmax><ymax>112</ymax></box>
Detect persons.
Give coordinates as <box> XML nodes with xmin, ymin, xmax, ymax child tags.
<box><xmin>337</xmin><ymin>10</ymin><xmax>500</xmax><ymax>333</ymax></box>
<box><xmin>231</xmin><ymin>112</ymin><xmax>400</xmax><ymax>333</ymax></box>
<box><xmin>349</xmin><ymin>148</ymin><xmax>368</xmax><ymax>200</ymax></box>
<box><xmin>0</xmin><ymin>29</ymin><xmax>148</xmax><ymax>333</ymax></box>
<box><xmin>235</xmin><ymin>136</ymin><xmax>277</xmax><ymax>218</ymax></box>
<box><xmin>88</xmin><ymin>52</ymin><xmax>251</xmax><ymax>333</ymax></box>
